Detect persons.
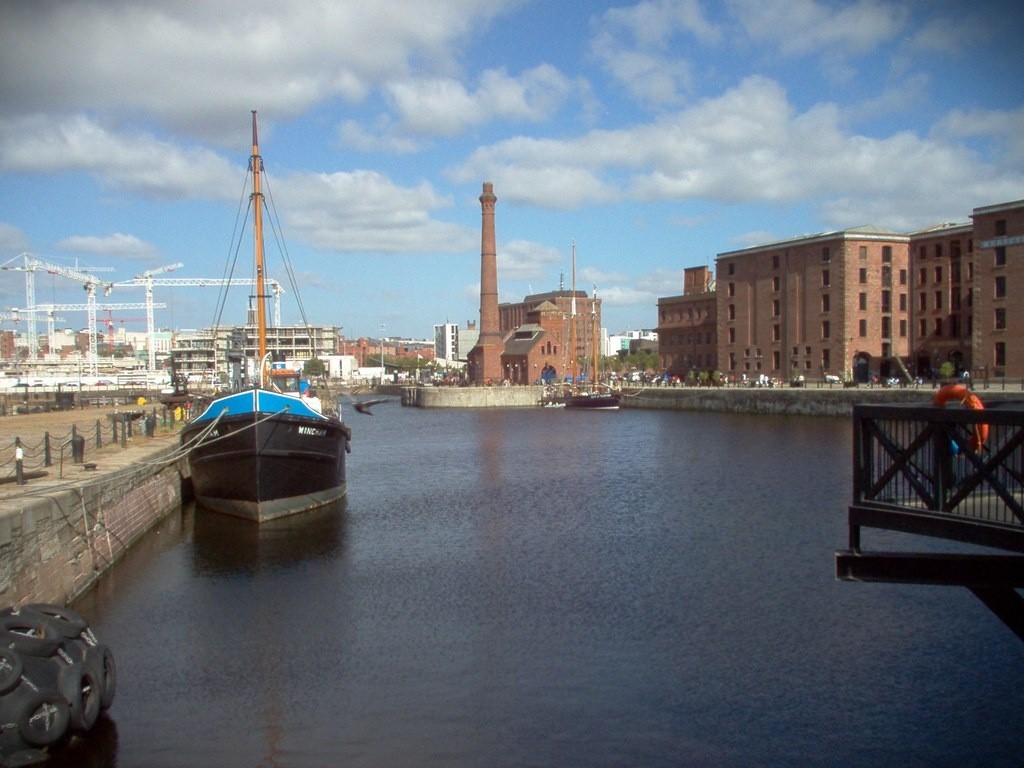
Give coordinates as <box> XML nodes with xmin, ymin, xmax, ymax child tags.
<box><xmin>718</xmin><ymin>372</ymin><xmax>778</xmax><ymax>388</ymax></box>
<box><xmin>866</xmin><ymin>376</ymin><xmax>922</xmax><ymax>389</ymax></box>
<box><xmin>608</xmin><ymin>374</ymin><xmax>699</xmax><ymax>388</ymax></box>
<box><xmin>963</xmin><ymin>371</ymin><xmax>975</xmax><ymax>390</ymax></box>
<box><xmin>397</xmin><ymin>376</ymin><xmax>515</xmax><ymax>388</ymax></box>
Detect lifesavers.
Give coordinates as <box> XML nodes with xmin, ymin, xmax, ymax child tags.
<box><xmin>930</xmin><ymin>384</ymin><xmax>991</xmax><ymax>460</ymax></box>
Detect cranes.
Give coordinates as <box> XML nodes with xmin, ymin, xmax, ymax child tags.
<box><xmin>0</xmin><ymin>253</ymin><xmax>184</xmax><ymax>377</ymax></box>
<box><xmin>113</xmin><ymin>262</ymin><xmax>276</xmax><ymax>374</ymax></box>
<box><xmin>271</xmin><ymin>279</ymin><xmax>285</xmax><ymax>326</ymax></box>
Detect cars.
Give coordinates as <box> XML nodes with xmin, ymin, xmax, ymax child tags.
<box><xmin>887</xmin><ymin>376</ymin><xmax>900</xmax><ymax>385</ymax></box>
<box><xmin>12</xmin><ymin>379</ymin><xmax>187</xmax><ymax>387</ymax></box>
<box><xmin>610</xmin><ymin>375</ymin><xmax>627</xmax><ymax>381</ymax></box>
<box><xmin>160</xmin><ymin>384</ymin><xmax>182</xmax><ymax>393</ymax></box>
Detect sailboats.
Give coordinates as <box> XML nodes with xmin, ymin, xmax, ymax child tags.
<box><xmin>178</xmin><ymin>110</ymin><xmax>354</xmax><ymax>524</ymax></box>
<box><xmin>537</xmin><ymin>239</ymin><xmax>620</xmax><ymax>410</ymax></box>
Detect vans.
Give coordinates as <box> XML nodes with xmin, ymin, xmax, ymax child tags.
<box><xmin>628</xmin><ymin>372</ymin><xmax>652</xmax><ymax>382</ymax></box>
